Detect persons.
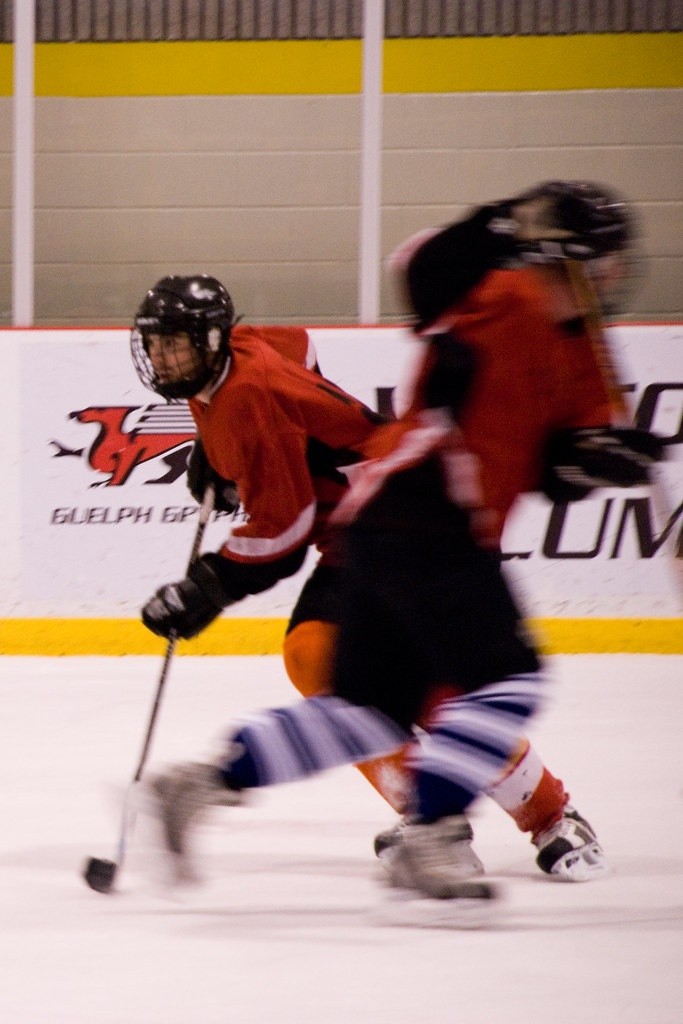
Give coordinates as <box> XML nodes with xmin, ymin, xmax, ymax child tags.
<box><xmin>131</xmin><ymin>179</ymin><xmax>668</xmax><ymax>897</ymax></box>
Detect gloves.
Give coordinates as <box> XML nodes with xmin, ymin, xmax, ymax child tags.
<box><xmin>187</xmin><ymin>434</ymin><xmax>240</xmax><ymax>514</ymax></box>
<box><xmin>141</xmin><ymin>559</ymin><xmax>235</xmax><ymax>640</ymax></box>
<box><xmin>533</xmin><ymin>414</ymin><xmax>665</xmax><ymax>503</ymax></box>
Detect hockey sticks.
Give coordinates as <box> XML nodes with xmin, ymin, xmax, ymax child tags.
<box><xmin>80</xmin><ymin>485</ymin><xmax>217</xmax><ymax>894</ymax></box>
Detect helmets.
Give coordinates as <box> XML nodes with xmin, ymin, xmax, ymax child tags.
<box><xmin>136</xmin><ymin>274</ymin><xmax>234</xmax><ymax>399</ymax></box>
<box><xmin>478</xmin><ymin>176</ymin><xmax>641</xmax><ymax>263</ymax></box>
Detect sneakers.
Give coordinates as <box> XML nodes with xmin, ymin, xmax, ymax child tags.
<box><xmin>397</xmin><ymin>813</ymin><xmax>491</xmax><ymax>900</ymax></box>
<box><xmin>150</xmin><ymin>757</ymin><xmax>241</xmax><ymax>890</ymax></box>
<box><xmin>533</xmin><ymin>803</ymin><xmax>606</xmax><ymax>882</ymax></box>
<box><xmin>373</xmin><ymin>817</ymin><xmax>476</xmax><ymax>867</ymax></box>
